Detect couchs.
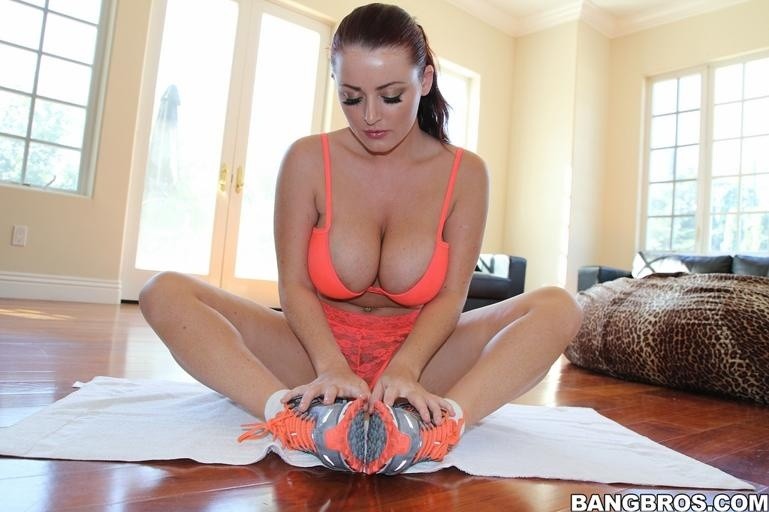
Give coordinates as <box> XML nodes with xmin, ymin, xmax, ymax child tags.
<box><xmin>575</xmin><ymin>251</ymin><xmax>769</xmax><ymax>296</ymax></box>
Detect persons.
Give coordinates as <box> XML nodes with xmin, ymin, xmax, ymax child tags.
<box><xmin>140</xmin><ymin>3</ymin><xmax>586</xmax><ymax>478</ymax></box>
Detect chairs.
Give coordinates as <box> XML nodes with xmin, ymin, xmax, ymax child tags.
<box><xmin>460</xmin><ymin>250</ymin><xmax>527</xmax><ymax>313</ymax></box>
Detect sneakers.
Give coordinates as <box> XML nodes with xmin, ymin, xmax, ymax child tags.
<box><xmin>366</xmin><ymin>400</ymin><xmax>463</xmax><ymax>477</ymax></box>
<box><xmin>238</xmin><ymin>396</ymin><xmax>364</xmax><ymax>477</ymax></box>
